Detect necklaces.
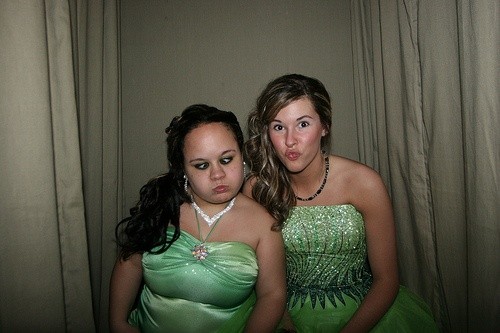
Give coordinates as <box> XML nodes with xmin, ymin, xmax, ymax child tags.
<box><xmin>290</xmin><ymin>150</ymin><xmax>330</xmax><ymax>201</ymax></box>
<box><xmin>190</xmin><ymin>195</ymin><xmax>236</xmax><ymax>226</ymax></box>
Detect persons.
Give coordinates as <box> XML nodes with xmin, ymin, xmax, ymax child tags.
<box><xmin>240</xmin><ymin>75</ymin><xmax>441</xmax><ymax>333</ymax></box>
<box><xmin>109</xmin><ymin>104</ymin><xmax>289</xmax><ymax>333</ymax></box>
